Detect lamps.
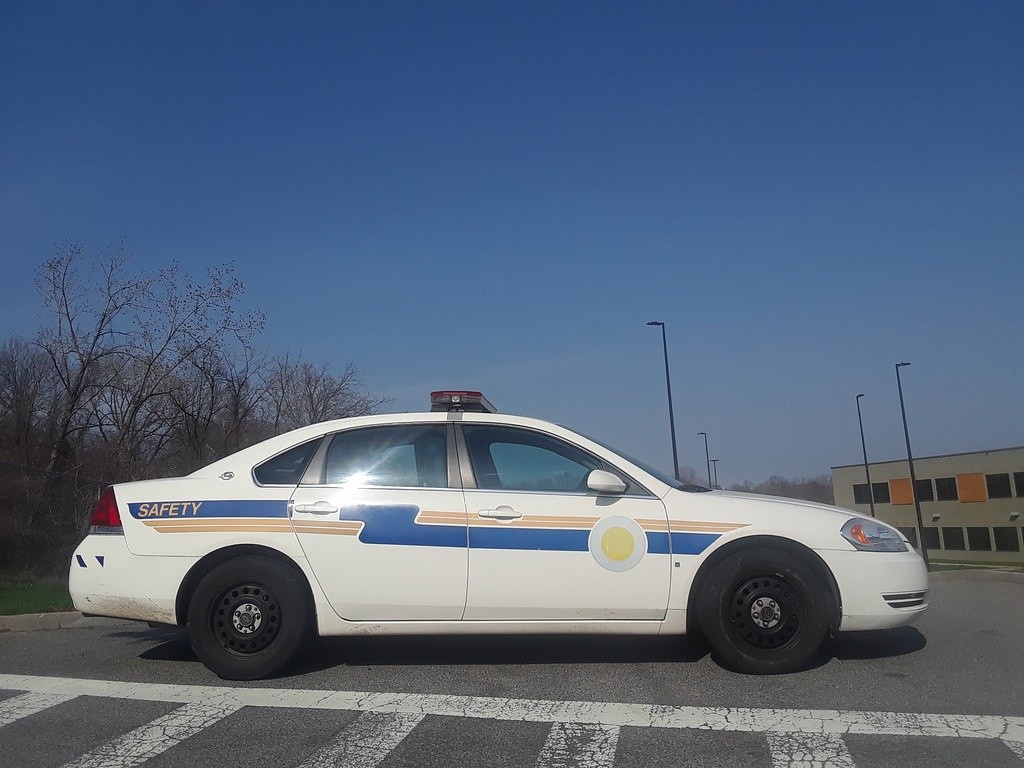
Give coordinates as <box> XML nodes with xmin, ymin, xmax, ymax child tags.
<box><xmin>1010</xmin><ymin>511</ymin><xmax>1021</xmax><ymax>517</ymax></box>
<box><xmin>932</xmin><ymin>513</ymin><xmax>941</xmax><ymax>518</ymax></box>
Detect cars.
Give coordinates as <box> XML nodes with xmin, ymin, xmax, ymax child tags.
<box><xmin>65</xmin><ymin>389</ymin><xmax>935</xmax><ymax>682</ymax></box>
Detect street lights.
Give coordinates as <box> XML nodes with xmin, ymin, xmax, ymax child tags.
<box><xmin>855</xmin><ymin>392</ymin><xmax>875</xmax><ymax>518</ymax></box>
<box><xmin>895</xmin><ymin>362</ymin><xmax>929</xmax><ymax>572</ymax></box>
<box><xmin>696</xmin><ymin>431</ymin><xmax>712</xmax><ymax>489</ymax></box>
<box><xmin>710</xmin><ymin>460</ymin><xmax>721</xmax><ymax>486</ymax></box>
<box><xmin>646</xmin><ymin>320</ymin><xmax>681</xmax><ymax>482</ymax></box>
